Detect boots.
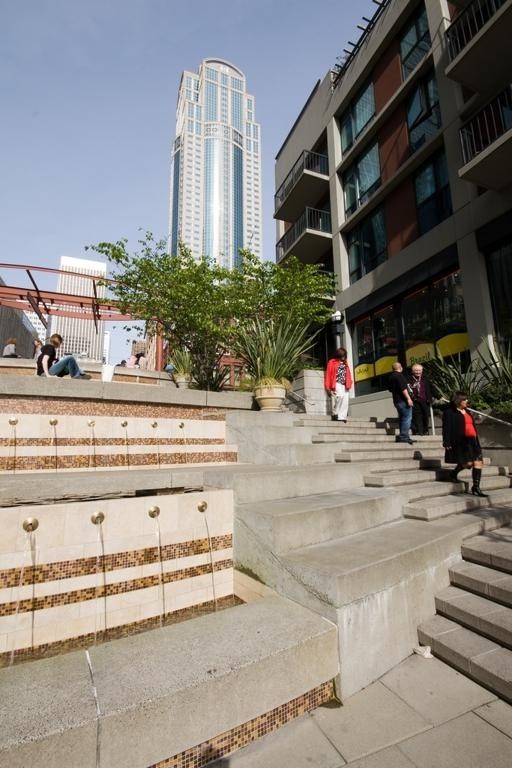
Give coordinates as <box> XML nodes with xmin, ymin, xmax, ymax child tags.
<box><xmin>472</xmin><ymin>468</ymin><xmax>489</xmax><ymax>496</ymax></box>
<box><xmin>448</xmin><ymin>464</ymin><xmax>464</xmax><ymax>483</ymax></box>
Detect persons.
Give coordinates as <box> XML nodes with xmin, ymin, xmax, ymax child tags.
<box><xmin>126</xmin><ymin>353</ymin><xmax>140</xmax><ymax>368</ymax></box>
<box><xmin>408</xmin><ymin>363</ymin><xmax>434</xmax><ymax>436</ymax></box>
<box><xmin>441</xmin><ymin>390</ymin><xmax>490</xmax><ymax>498</ymax></box>
<box><xmin>325</xmin><ymin>347</ymin><xmax>353</xmax><ymax>422</ymax></box>
<box><xmin>386</xmin><ymin>360</ymin><xmax>418</xmax><ymax>445</ymax></box>
<box><xmin>2</xmin><ymin>337</ymin><xmax>22</xmax><ymax>359</ymax></box>
<box><xmin>35</xmin><ymin>334</ymin><xmax>92</xmax><ymax>381</ymax></box>
<box><xmin>32</xmin><ymin>338</ymin><xmax>44</xmax><ymax>359</ymax></box>
<box><xmin>138</xmin><ymin>352</ymin><xmax>148</xmax><ymax>370</ymax></box>
<box><xmin>115</xmin><ymin>360</ymin><xmax>127</xmax><ymax>367</ymax></box>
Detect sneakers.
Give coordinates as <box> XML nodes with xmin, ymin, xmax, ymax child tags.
<box><xmin>77</xmin><ymin>374</ymin><xmax>91</xmax><ymax>380</ymax></box>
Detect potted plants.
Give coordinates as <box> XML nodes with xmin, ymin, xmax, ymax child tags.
<box><xmin>166</xmin><ymin>347</ymin><xmax>195</xmax><ymax>389</ymax></box>
<box><xmin>223</xmin><ymin>312</ymin><xmax>326</xmax><ymax>412</ymax></box>
